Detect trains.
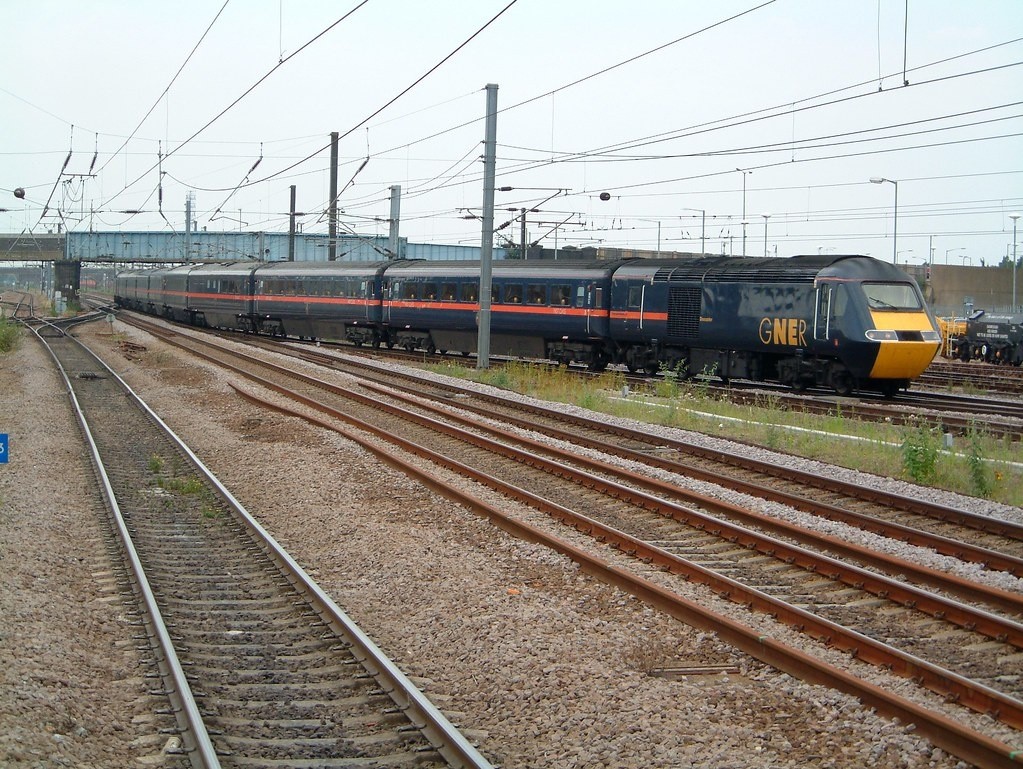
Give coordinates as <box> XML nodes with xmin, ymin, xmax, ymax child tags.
<box><xmin>113</xmin><ymin>259</ymin><xmax>942</xmax><ymax>399</ymax></box>
<box><xmin>957</xmin><ymin>307</ymin><xmax>1023</xmax><ymax>368</ymax></box>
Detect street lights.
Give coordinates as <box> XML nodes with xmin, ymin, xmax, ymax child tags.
<box><xmin>764</xmin><ymin>214</ymin><xmax>1010</xmax><ymax>266</ymax></box>
<box><xmin>870</xmin><ymin>177</ymin><xmax>897</xmax><ymax>266</ymax></box>
<box><xmin>640</xmin><ymin>219</ymin><xmax>661</xmax><ymax>260</ymax></box>
<box><xmin>1010</xmin><ymin>214</ymin><xmax>1022</xmax><ymax>311</ymax></box>
<box><xmin>735</xmin><ymin>166</ymin><xmax>751</xmax><ymax>257</ymax></box>
<box><xmin>684</xmin><ymin>207</ymin><xmax>706</xmax><ymax>259</ymax></box>
